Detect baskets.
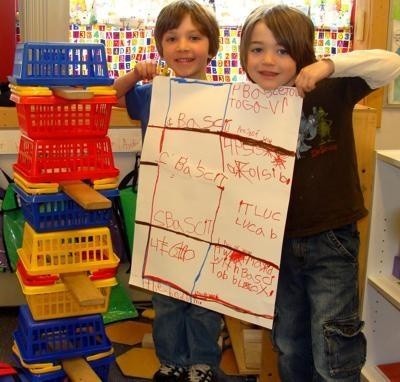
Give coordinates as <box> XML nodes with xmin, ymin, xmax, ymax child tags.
<box><xmin>8</xmin><ymin>42</ymin><xmax>120</xmax><ymax>381</ymax></box>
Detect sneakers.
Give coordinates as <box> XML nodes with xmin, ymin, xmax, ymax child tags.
<box><xmin>153</xmin><ymin>362</ymin><xmax>214</xmax><ymax>382</ymax></box>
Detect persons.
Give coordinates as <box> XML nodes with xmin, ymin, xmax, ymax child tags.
<box><xmin>110</xmin><ymin>0</ymin><xmax>224</xmax><ymax>382</ymax></box>
<box><xmin>238</xmin><ymin>2</ymin><xmax>400</xmax><ymax>381</ymax></box>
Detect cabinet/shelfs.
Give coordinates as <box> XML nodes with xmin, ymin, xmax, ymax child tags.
<box><xmin>359</xmin><ymin>149</ymin><xmax>400</xmax><ymax>381</ymax></box>
